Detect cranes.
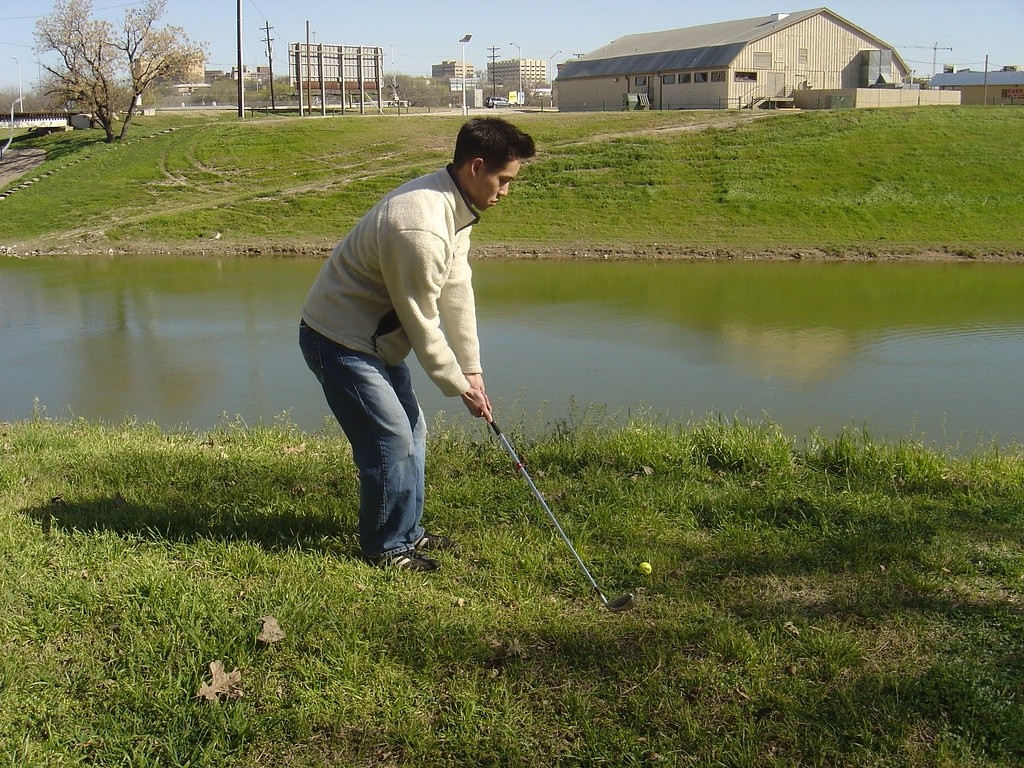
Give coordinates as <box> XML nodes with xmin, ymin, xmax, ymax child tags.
<box><xmin>898</xmin><ymin>41</ymin><xmax>952</xmax><ymax>76</ymax></box>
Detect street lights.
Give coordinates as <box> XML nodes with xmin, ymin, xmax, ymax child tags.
<box><xmin>12</xmin><ymin>56</ymin><xmax>24</xmax><ymax>113</ymax></box>
<box><xmin>510</xmin><ymin>42</ymin><xmax>522</xmax><ymax>107</ymax></box>
<box><xmin>459</xmin><ymin>33</ymin><xmax>472</xmax><ymax>115</ymax></box>
<box><xmin>550</xmin><ymin>50</ymin><xmax>563</xmax><ymax>107</ymax></box>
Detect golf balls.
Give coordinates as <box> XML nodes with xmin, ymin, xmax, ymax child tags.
<box><xmin>638</xmin><ymin>561</ymin><xmax>652</xmax><ymax>577</ymax></box>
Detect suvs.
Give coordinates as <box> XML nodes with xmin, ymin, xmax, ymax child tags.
<box><xmin>485</xmin><ymin>97</ymin><xmax>515</xmax><ymax>109</ymax></box>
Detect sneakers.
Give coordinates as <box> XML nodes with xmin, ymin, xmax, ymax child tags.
<box><xmin>365</xmin><ymin>550</ymin><xmax>441</xmax><ymax>573</ymax></box>
<box><xmin>405</xmin><ymin>531</ymin><xmax>451</xmax><ymax>552</ymax></box>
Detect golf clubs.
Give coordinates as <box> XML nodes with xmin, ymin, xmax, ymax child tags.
<box><xmin>489</xmin><ymin>419</ymin><xmax>634</xmax><ymax>612</ymax></box>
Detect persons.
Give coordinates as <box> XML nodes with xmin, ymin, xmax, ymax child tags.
<box><xmin>298</xmin><ymin>116</ymin><xmax>536</xmax><ymax>572</ymax></box>
